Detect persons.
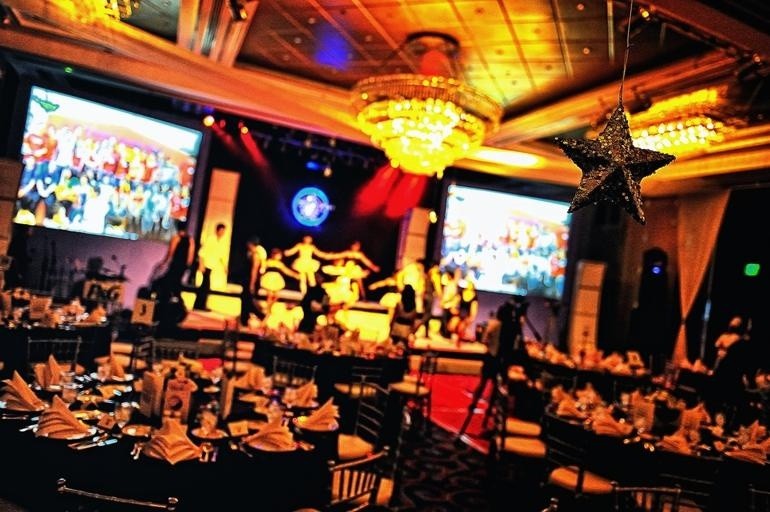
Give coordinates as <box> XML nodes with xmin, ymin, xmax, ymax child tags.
<box><xmin>702</xmin><ymin>312</ymin><xmax>770</xmax><ymax>405</ymax></box>
<box><xmin>142</xmin><ymin>216</ymin><xmax>568</xmax><ymax>416</ymax></box>
<box><xmin>15</xmin><ymin>109</ymin><xmax>197</xmax><ymax>242</ymax></box>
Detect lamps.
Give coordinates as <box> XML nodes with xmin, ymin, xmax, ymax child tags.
<box><xmin>344</xmin><ymin>32</ymin><xmax>508</xmax><ymax>180</ymax></box>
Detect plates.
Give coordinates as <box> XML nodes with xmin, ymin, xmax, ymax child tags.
<box><xmin>521</xmin><ymin>338</ymin><xmax>650</xmax><ymax>377</ymax></box>
<box><xmin>240</xmin><ymin>314</ymin><xmax>407</xmax><ymax>359</ymax></box>
<box><xmin>0</xmin><ymin>288</ymin><xmax>109</xmax><ymax>330</ymax></box>
<box><xmin>0</xmin><ymin>350</ymin><xmax>341</xmax><ymax>465</ymax></box>
<box><xmin>551</xmin><ymin>383</ymin><xmax>770</xmax><ymax>466</ymax></box>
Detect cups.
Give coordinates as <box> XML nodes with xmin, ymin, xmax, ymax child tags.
<box><xmin>663</xmin><ymin>362</ymin><xmax>679</xmax><ymax>389</ymax></box>
